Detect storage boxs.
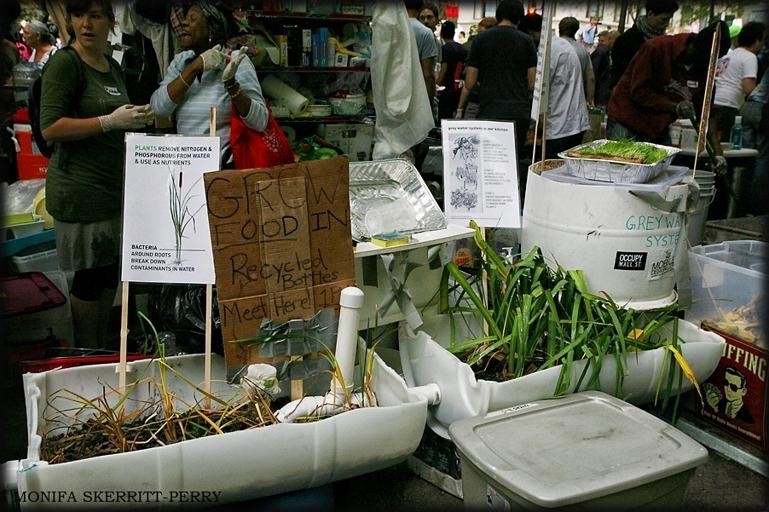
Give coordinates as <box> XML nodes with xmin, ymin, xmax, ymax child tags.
<box><xmin>0</xmin><ymin>271</ymin><xmax>77</xmax><ymax>365</ymax></box>
<box><xmin>318</xmin><ymin>123</ymin><xmax>374</xmax><ymax>162</ymax></box>
<box><xmin>450</xmin><ymin>391</ymin><xmax>709</xmax><ymax>512</ymax></box>
<box><xmin>686</xmin><ymin>240</ymin><xmax>769</xmax><ymax>353</ymax></box>
<box><xmin>12</xmin><ymin>122</ymin><xmax>48</xmax><ymax>181</ymax></box>
<box><xmin>694</xmin><ymin>323</ymin><xmax>768</xmax><ymax>452</ymax></box>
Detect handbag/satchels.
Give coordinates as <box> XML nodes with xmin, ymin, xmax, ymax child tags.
<box><xmin>28</xmin><ymin>45</ymin><xmax>85</xmax><ymax>159</ymax></box>
<box><xmin>230</xmin><ymin>95</ymin><xmax>294</xmax><ymax>169</ymax></box>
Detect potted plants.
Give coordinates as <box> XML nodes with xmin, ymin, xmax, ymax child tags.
<box><xmin>402</xmin><ymin>219</ymin><xmax>725</xmax><ymax>430</ymax></box>
<box><xmin>557</xmin><ymin>126</ymin><xmax>683</xmax><ymax>185</ymax></box>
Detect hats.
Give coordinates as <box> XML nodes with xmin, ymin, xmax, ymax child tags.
<box><xmin>697</xmin><ymin>20</ymin><xmax>731</xmax><ymax>54</ymax></box>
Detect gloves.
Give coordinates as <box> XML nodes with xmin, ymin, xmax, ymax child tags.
<box><xmin>710</xmin><ymin>155</ymin><xmax>728</xmax><ymax>177</ymax></box>
<box><xmin>133</xmin><ymin>104</ymin><xmax>155</xmax><ymax>125</ymax></box>
<box><xmin>675</xmin><ymin>101</ymin><xmax>697</xmax><ymax>122</ymax></box>
<box><xmin>98</xmin><ymin>104</ymin><xmax>145</xmax><ymax>133</ymax></box>
<box><xmin>455</xmin><ymin>110</ymin><xmax>464</xmax><ymax>119</ymax></box>
<box><xmin>200</xmin><ymin>44</ymin><xmax>231</xmax><ymax>74</ymax></box>
<box><xmin>221</xmin><ymin>46</ymin><xmax>249</xmax><ymax>83</ymax></box>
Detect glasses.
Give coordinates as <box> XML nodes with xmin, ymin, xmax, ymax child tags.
<box><xmin>722</xmin><ymin>379</ymin><xmax>742</xmax><ymax>391</ymax></box>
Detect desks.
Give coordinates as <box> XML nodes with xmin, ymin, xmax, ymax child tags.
<box><xmin>677</xmin><ymin>145</ymin><xmax>759</xmax><ymax>219</ymax></box>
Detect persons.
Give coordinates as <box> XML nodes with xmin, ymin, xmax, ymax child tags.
<box><xmin>437</xmin><ymin>21</ymin><xmax>462</xmax><ymax>129</ymax></box>
<box><xmin>521</xmin><ymin>13</ymin><xmax>591</xmax><ymax>159</ymax></box>
<box><xmin>455</xmin><ymin>0</ymin><xmax>537</xmax><ymax>149</ymax></box>
<box><xmin>705</xmin><ymin>366</ymin><xmax>755</xmax><ymax>425</ymax></box>
<box><xmin>40</xmin><ymin>1</ymin><xmax>154</xmax><ymax>353</ymax></box>
<box><xmin>583</xmin><ymin>17</ymin><xmax>598</xmax><ymax>46</ymax></box>
<box><xmin>476</xmin><ymin>17</ymin><xmax>496</xmax><ymax>34</ymax></box>
<box><xmin>612</xmin><ymin>0</ymin><xmax>678</xmax><ymax>86</ymax></box>
<box><xmin>606</xmin><ymin>19</ymin><xmax>730</xmax><ymax>144</ymax></box>
<box><xmin>402</xmin><ymin>0</ymin><xmax>436</xmax><ymax>172</ymax></box>
<box><xmin>590</xmin><ymin>30</ymin><xmax>621</xmax><ymax>106</ymax></box>
<box><xmin>712</xmin><ymin>21</ymin><xmax>765</xmax><ymax>140</ymax></box>
<box><xmin>14</xmin><ymin>17</ymin><xmax>57</xmax><ymax>63</ymax></box>
<box><xmin>418</xmin><ymin>7</ymin><xmax>442</xmax><ymax>66</ymax></box>
<box><xmin>555</xmin><ymin>16</ymin><xmax>596</xmax><ymax>111</ymax></box>
<box><xmin>148</xmin><ymin>3</ymin><xmax>270</xmax><ymax>171</ymax></box>
<box><xmin>598</xmin><ymin>30</ymin><xmax>609</xmax><ymax>48</ymax></box>
<box><xmin>740</xmin><ymin>67</ymin><xmax>768</xmax><ymax>137</ymax></box>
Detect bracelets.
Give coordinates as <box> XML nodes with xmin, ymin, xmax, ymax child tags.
<box><xmin>178</xmin><ymin>72</ymin><xmax>191</xmax><ymax>89</ymax></box>
<box><xmin>223</xmin><ymin>79</ymin><xmax>237</xmax><ymax>89</ymax></box>
<box><xmin>230</xmin><ymin>88</ymin><xmax>241</xmax><ymax>98</ymax></box>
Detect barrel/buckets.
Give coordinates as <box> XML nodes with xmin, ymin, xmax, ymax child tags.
<box><xmin>686</xmin><ymin>169</ymin><xmax>716</xmax><ymax>246</ymax></box>
<box><xmin>520</xmin><ymin>159</ymin><xmax>690</xmax><ymax>310</ymax></box>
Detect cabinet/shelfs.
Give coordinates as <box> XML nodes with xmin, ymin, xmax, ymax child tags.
<box><xmin>234</xmin><ymin>0</ymin><xmax>374</xmax><ymax>160</ymax></box>
